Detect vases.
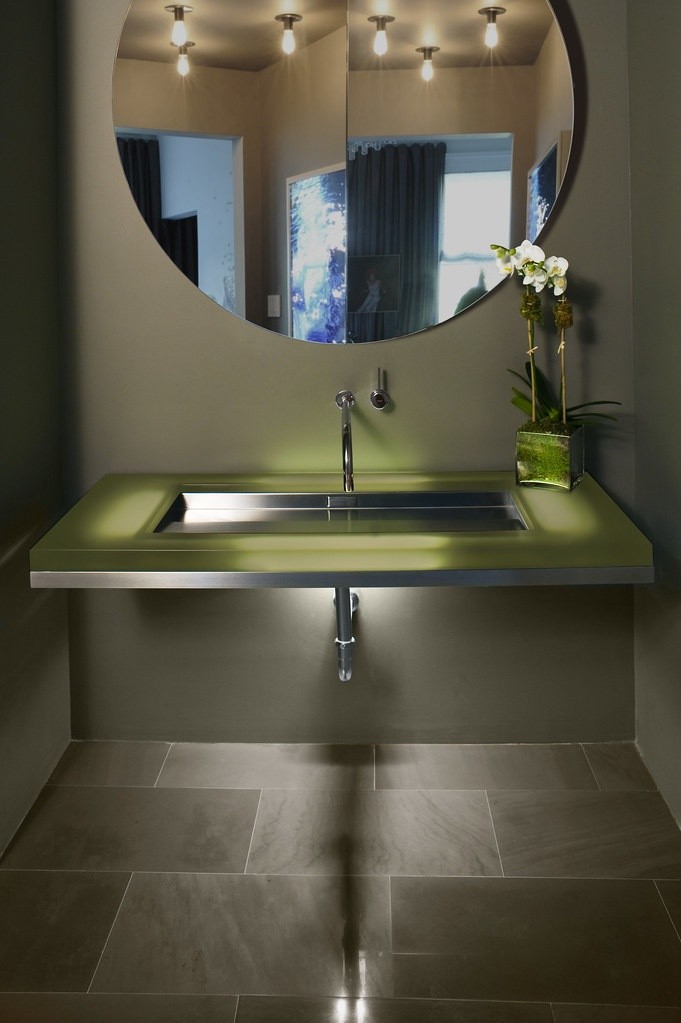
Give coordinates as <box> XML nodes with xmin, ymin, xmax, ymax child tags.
<box><xmin>515</xmin><ymin>418</ymin><xmax>585</xmax><ymax>490</ymax></box>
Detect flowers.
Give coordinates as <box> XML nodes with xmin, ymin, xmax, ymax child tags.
<box><xmin>490</xmin><ymin>238</ymin><xmax>570</xmax><ymax>424</ymax></box>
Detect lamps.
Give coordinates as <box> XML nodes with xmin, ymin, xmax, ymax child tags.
<box><xmin>478</xmin><ymin>7</ymin><xmax>507</xmax><ymax>48</ymax></box>
<box><xmin>164</xmin><ymin>4</ymin><xmax>196</xmax><ymax>77</ymax></box>
<box><xmin>275</xmin><ymin>14</ymin><xmax>303</xmax><ymax>55</ymax></box>
<box><xmin>414</xmin><ymin>47</ymin><xmax>441</xmax><ymax>81</ymax></box>
<box><xmin>367</xmin><ymin>15</ymin><xmax>395</xmax><ymax>56</ymax></box>
<box><xmin>267</xmin><ymin>295</ymin><xmax>281</xmax><ymax>318</ymax></box>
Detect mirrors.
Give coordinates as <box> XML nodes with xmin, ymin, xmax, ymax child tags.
<box><xmin>111</xmin><ymin>0</ymin><xmax>574</xmax><ymax>348</ymax></box>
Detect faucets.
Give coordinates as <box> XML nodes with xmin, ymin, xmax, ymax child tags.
<box><xmin>334</xmin><ymin>389</ymin><xmax>357</xmax><ymax>494</ymax></box>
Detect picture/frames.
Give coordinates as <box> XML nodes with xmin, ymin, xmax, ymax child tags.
<box><xmin>346</xmin><ymin>254</ymin><xmax>401</xmax><ymax>314</ymax></box>
<box><xmin>525</xmin><ymin>130</ymin><xmax>572</xmax><ymax>244</ymax></box>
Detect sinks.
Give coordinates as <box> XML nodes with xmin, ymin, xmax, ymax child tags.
<box><xmin>132</xmin><ymin>488</ymin><xmax>541</xmax><ymax>542</ymax></box>
